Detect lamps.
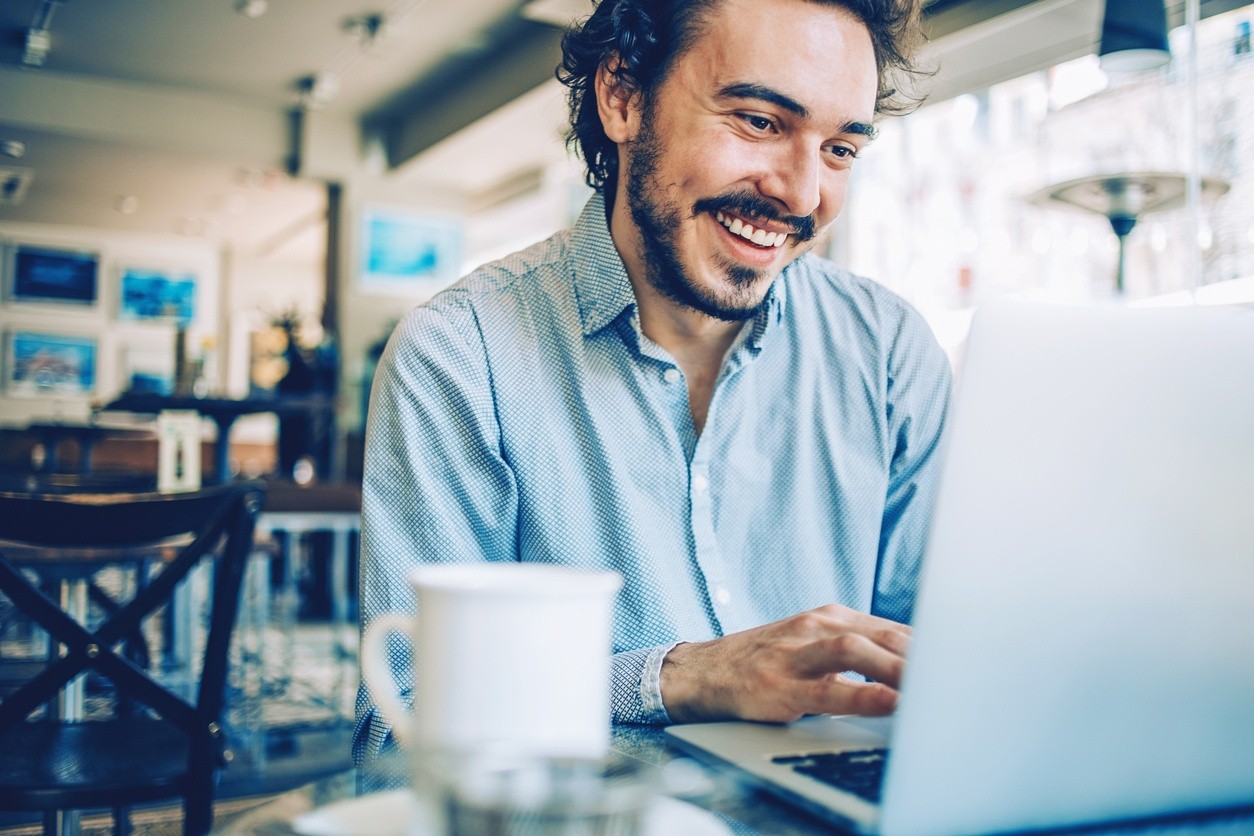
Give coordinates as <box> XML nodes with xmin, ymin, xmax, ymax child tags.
<box><xmin>1027</xmin><ymin>85</ymin><xmax>1232</xmax><ymax>295</ymax></box>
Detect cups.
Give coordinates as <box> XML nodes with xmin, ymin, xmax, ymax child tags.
<box><xmin>357</xmin><ymin>561</ymin><xmax>622</xmax><ymax>807</ymax></box>
<box><xmin>159</xmin><ymin>410</ymin><xmax>205</xmax><ymax>496</ymax></box>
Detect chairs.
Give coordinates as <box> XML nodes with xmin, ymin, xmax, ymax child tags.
<box><xmin>0</xmin><ymin>421</ymin><xmax>362</xmax><ymax>836</ymax></box>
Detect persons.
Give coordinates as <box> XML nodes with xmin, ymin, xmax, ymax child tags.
<box><xmin>271</xmin><ymin>296</ymin><xmax>341</xmax><ymax>482</ymax></box>
<box><xmin>349</xmin><ymin>0</ymin><xmax>953</xmax><ymax>799</ymax></box>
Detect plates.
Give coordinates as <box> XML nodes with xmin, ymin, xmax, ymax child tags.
<box><xmin>289</xmin><ymin>782</ymin><xmax>735</xmax><ymax>836</ymax></box>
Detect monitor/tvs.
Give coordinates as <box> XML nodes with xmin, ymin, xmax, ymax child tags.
<box><xmin>2</xmin><ymin>243</ymin><xmax>101</xmax><ymax>307</ymax></box>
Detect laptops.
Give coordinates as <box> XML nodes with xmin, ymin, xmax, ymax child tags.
<box><xmin>665</xmin><ymin>301</ymin><xmax>1253</xmax><ymax>835</ymax></box>
